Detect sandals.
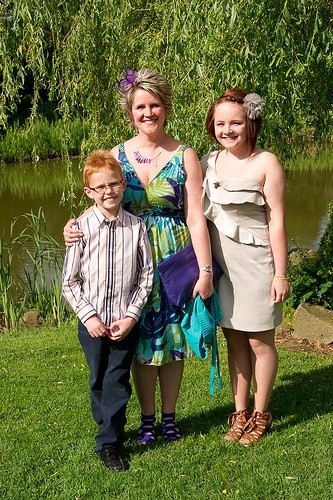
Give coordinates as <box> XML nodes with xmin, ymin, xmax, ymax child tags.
<box><xmin>224</xmin><ymin>408</ymin><xmax>250</xmax><ymax>443</ymax></box>
<box><xmin>162</xmin><ymin>412</ymin><xmax>182</xmax><ymax>441</ymax></box>
<box><xmin>136</xmin><ymin>411</ymin><xmax>157</xmax><ymax>446</ymax></box>
<box><xmin>239</xmin><ymin>407</ymin><xmax>272</xmax><ymax>447</ymax></box>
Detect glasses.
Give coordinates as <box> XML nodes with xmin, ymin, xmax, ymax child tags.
<box><xmin>89</xmin><ymin>180</ymin><xmax>122</xmax><ymax>194</ymax></box>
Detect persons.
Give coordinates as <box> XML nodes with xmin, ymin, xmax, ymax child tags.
<box><xmin>200</xmin><ymin>89</ymin><xmax>293</xmax><ymax>446</ymax></box>
<box><xmin>61</xmin><ymin>150</ymin><xmax>154</xmax><ymax>471</ymax></box>
<box><xmin>63</xmin><ymin>67</ymin><xmax>214</xmax><ymax>444</ymax></box>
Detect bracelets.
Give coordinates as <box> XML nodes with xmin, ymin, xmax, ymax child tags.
<box><xmin>274</xmin><ymin>275</ymin><xmax>288</xmax><ymax>280</ymax></box>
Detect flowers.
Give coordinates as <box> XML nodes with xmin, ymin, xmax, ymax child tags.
<box><xmin>119</xmin><ymin>65</ymin><xmax>137</xmax><ymax>85</ymax></box>
<box><xmin>244</xmin><ymin>93</ymin><xmax>266</xmax><ymax>119</ymax></box>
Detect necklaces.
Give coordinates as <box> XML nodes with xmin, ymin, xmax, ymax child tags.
<box><xmin>133</xmin><ymin>132</ymin><xmax>167</xmax><ymax>164</ymax></box>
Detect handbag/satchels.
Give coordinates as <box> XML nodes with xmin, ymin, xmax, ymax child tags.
<box><xmin>158</xmin><ymin>244</ymin><xmax>224</xmax><ymax>308</ymax></box>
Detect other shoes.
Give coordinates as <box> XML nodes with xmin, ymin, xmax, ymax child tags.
<box><xmin>100</xmin><ymin>447</ymin><xmax>126</xmax><ymax>472</ymax></box>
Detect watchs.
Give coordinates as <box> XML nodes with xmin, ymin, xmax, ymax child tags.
<box><xmin>199</xmin><ymin>265</ymin><xmax>213</xmax><ymax>272</ymax></box>
